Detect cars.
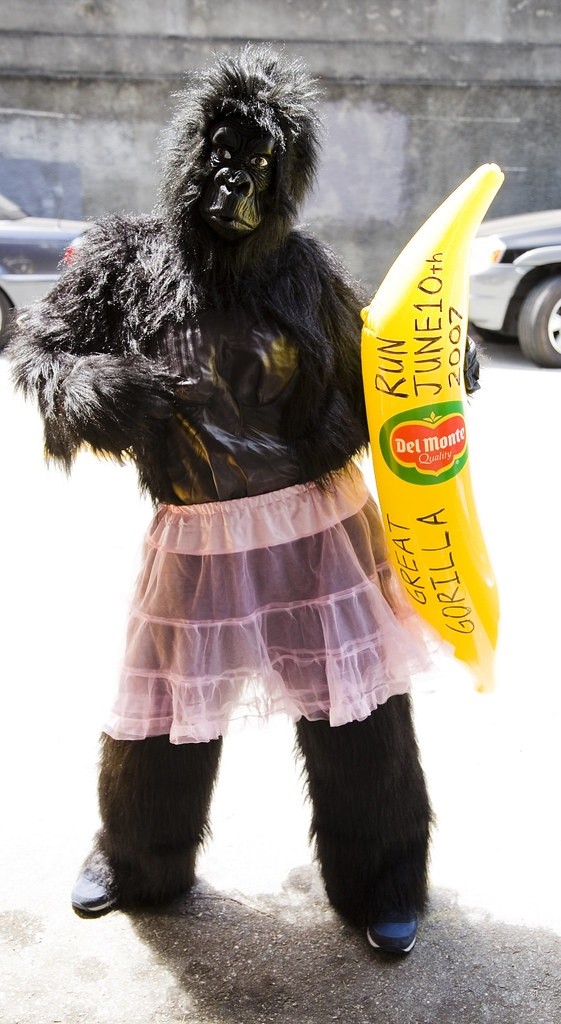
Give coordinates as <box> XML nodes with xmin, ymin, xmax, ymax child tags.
<box><xmin>0</xmin><ymin>192</ymin><xmax>102</xmax><ymax>354</ymax></box>
<box><xmin>465</xmin><ymin>206</ymin><xmax>561</xmax><ymax>366</ymax></box>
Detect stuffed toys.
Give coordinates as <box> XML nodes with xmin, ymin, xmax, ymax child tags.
<box><xmin>7</xmin><ymin>45</ymin><xmax>481</xmax><ymax>959</ymax></box>
